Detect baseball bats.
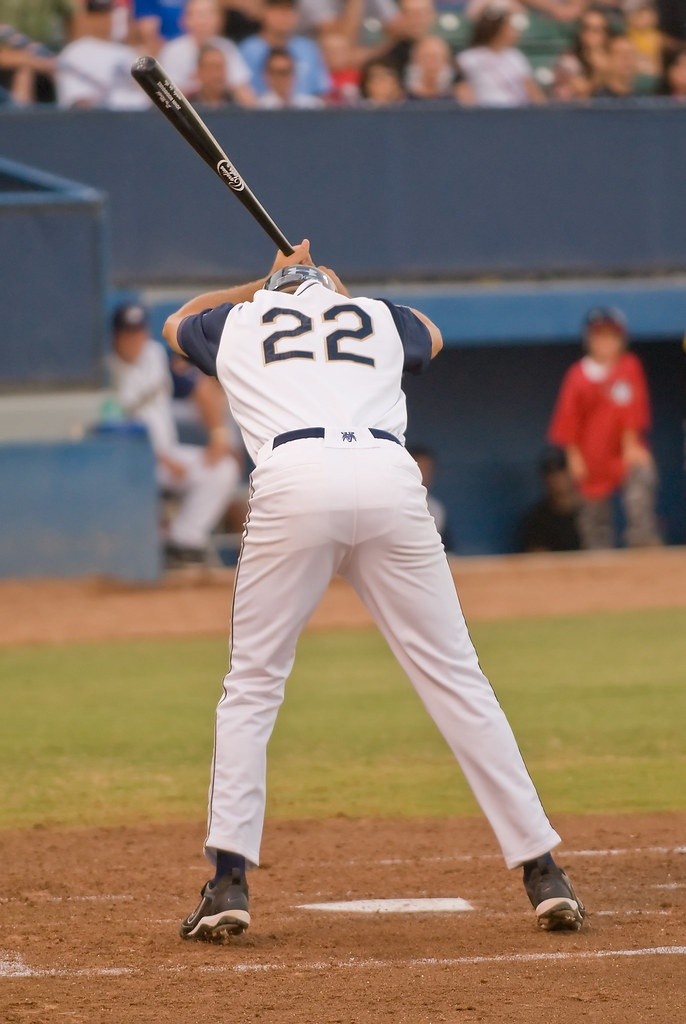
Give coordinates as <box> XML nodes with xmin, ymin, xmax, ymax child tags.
<box><xmin>126</xmin><ymin>51</ymin><xmax>301</xmax><ymax>261</ymax></box>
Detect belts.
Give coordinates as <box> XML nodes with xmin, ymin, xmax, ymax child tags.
<box><xmin>272</xmin><ymin>427</ymin><xmax>403</xmax><ymax>448</ymax></box>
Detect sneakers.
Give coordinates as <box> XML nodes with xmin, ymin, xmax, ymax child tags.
<box><xmin>522</xmin><ymin>859</ymin><xmax>585</xmax><ymax>932</ymax></box>
<box><xmin>180</xmin><ymin>867</ymin><xmax>250</xmax><ymax>943</ymax></box>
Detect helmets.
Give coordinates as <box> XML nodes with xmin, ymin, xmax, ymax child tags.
<box><xmin>113</xmin><ymin>300</ymin><xmax>151</xmax><ymax>336</ymax></box>
<box><xmin>584</xmin><ymin>304</ymin><xmax>630</xmax><ymax>347</ymax></box>
<box><xmin>263</xmin><ymin>264</ymin><xmax>337</xmax><ymax>296</ymax></box>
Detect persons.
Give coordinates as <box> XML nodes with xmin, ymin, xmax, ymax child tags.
<box><xmin>0</xmin><ymin>0</ymin><xmax>686</xmax><ymax>565</ymax></box>
<box><xmin>160</xmin><ymin>240</ymin><xmax>586</xmax><ymax>941</ymax></box>
